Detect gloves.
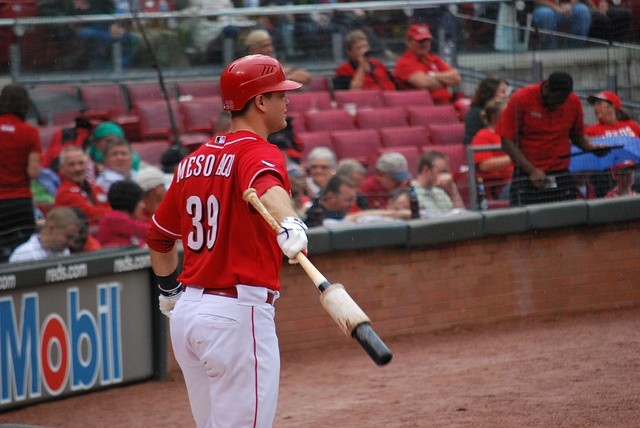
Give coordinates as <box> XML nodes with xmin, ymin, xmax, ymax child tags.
<box><xmin>276</xmin><ymin>218</ymin><xmax>307</xmax><ymax>264</ymax></box>
<box><xmin>158</xmin><ymin>284</ymin><xmax>183</xmax><ymax>318</ymax></box>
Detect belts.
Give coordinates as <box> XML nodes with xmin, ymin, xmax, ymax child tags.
<box><xmin>182</xmin><ymin>287</ymin><xmax>274</xmax><ymax>304</ymax></box>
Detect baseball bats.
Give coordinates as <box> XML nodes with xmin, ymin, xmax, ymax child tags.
<box><xmin>242</xmin><ymin>188</ymin><xmax>393</xmax><ymax>366</ymax></box>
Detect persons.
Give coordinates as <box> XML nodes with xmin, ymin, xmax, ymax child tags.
<box><xmin>465</xmin><ymin>78</ymin><xmax>508</xmax><ymax>150</ymax></box>
<box><xmin>10</xmin><ymin>204</ymin><xmax>81</xmax><ymax>263</ymax></box>
<box><xmin>499</xmin><ymin>71</ymin><xmax>610</xmax><ymax>209</ymax></box>
<box><xmin>146</xmin><ymin>53</ymin><xmax>308</xmax><ymax>427</ymax></box>
<box><xmin>132</xmin><ymin>165</ymin><xmax>166</xmax><ymax>219</ymax></box>
<box><xmin>1</xmin><ymin>84</ymin><xmax>43</xmax><ymax>263</ymax></box>
<box><xmin>335</xmin><ymin>159</ymin><xmax>367</xmax><ymax>186</ymax></box>
<box><xmin>526</xmin><ymin>1</ymin><xmax>636</xmax><ymax>52</ymax></box>
<box><xmin>608</xmin><ymin>158</ymin><xmax>638</xmax><ymax>197</ymax></box>
<box><xmin>362</xmin><ymin>151</ymin><xmax>413</xmax><ymax>217</ymax></box>
<box><xmin>71</xmin><ymin>206</ymin><xmax>101</xmax><ymax>254</ymax></box>
<box><xmin>470</xmin><ymin>98</ymin><xmax>513</xmax><ymax>205</ymax></box>
<box><xmin>2</xmin><ymin>1</ymin><xmax>524</xmax><ymax>60</ymax></box>
<box><xmin>249</xmin><ymin>28</ymin><xmax>312</xmax><ymax>84</ymax></box>
<box><xmin>393</xmin><ymin>23</ymin><xmax>461</xmax><ymax>104</ymax></box>
<box><xmin>90</xmin><ymin>121</ymin><xmax>147</xmax><ymax>172</ymax></box>
<box><xmin>334</xmin><ymin>29</ymin><xmax>400</xmax><ymax>91</ymax></box>
<box><xmin>96</xmin><ymin>138</ymin><xmax>140</xmax><ymax>189</ymax></box>
<box><xmin>304</xmin><ymin>176</ymin><xmax>359</xmax><ymax>226</ymax></box>
<box><xmin>305</xmin><ymin>146</ymin><xmax>337</xmax><ymax>197</ymax></box>
<box><xmin>582</xmin><ymin>90</ymin><xmax>639</xmax><ymax>140</ymax></box>
<box><xmin>55</xmin><ymin>145</ymin><xmax>111</xmax><ymax>220</ymax></box>
<box><xmin>391</xmin><ymin>150</ymin><xmax>467</xmax><ymax>221</ymax></box>
<box><xmin>98</xmin><ymin>181</ymin><xmax>152</xmax><ymax>247</ymax></box>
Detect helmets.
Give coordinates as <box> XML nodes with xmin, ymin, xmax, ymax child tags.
<box><xmin>221</xmin><ymin>54</ymin><xmax>303</xmax><ymax>110</ymax></box>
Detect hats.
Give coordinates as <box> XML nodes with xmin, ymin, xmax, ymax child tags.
<box><xmin>610</xmin><ymin>158</ymin><xmax>639</xmax><ymax>175</ymax></box>
<box><xmin>586</xmin><ymin>91</ymin><xmax>622</xmax><ymax>111</ymax></box>
<box><xmin>409</xmin><ymin>23</ymin><xmax>432</xmax><ymax>42</ymax></box>
<box><xmin>377</xmin><ymin>153</ymin><xmax>411</xmax><ymax>180</ymax></box>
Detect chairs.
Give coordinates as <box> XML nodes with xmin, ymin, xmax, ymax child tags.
<box><xmin>35</xmin><ymin>71</ymin><xmax>471</xmax><ymax>223</ymax></box>
